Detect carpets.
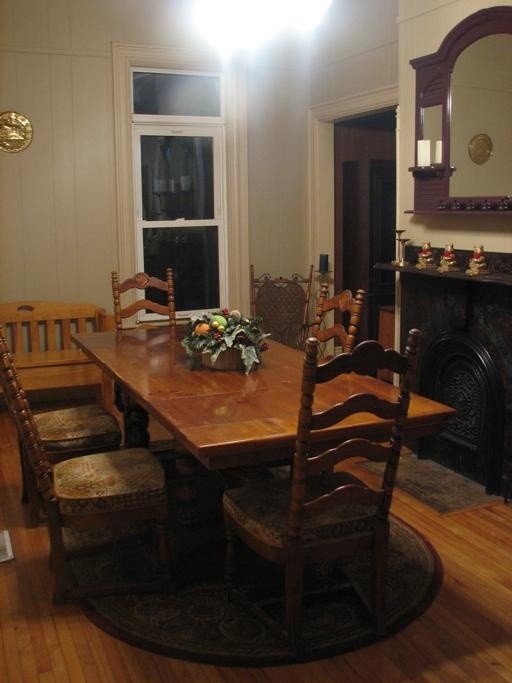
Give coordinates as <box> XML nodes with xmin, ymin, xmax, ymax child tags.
<box><xmin>354</xmin><ymin>450</ymin><xmax>504</xmax><ymax>519</ymax></box>
<box><xmin>54</xmin><ymin>437</ymin><xmax>443</xmax><ymax>669</ymax></box>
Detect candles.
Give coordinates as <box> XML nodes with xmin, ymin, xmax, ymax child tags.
<box><xmin>319</xmin><ymin>253</ymin><xmax>328</xmax><ymax>272</ymax></box>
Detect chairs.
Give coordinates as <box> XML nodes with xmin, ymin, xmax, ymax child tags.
<box><xmin>0</xmin><ymin>301</ymin><xmax>124</xmax><ymax>431</ymax></box>
<box><xmin>110</xmin><ymin>267</ymin><xmax>176</xmax><ymax>332</ymax></box>
<box><xmin>0</xmin><ymin>323</ymin><xmax>172</xmax><ymax>570</ymax></box>
<box><xmin>248</xmin><ymin>264</ymin><xmax>314</xmax><ymax>351</ymax></box>
<box><xmin>310</xmin><ymin>281</ymin><xmax>365</xmax><ymax>373</ymax></box>
<box><xmin>4</xmin><ymin>395</ymin><xmax>123</xmax><ymax>529</ymax></box>
<box><xmin>219</xmin><ymin>325</ymin><xmax>423</xmax><ymax>655</ymax></box>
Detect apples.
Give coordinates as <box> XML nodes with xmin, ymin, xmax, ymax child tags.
<box><xmin>211</xmin><ymin>314</ymin><xmax>228</xmax><ymax>327</ymax></box>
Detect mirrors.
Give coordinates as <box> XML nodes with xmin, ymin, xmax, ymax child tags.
<box><xmin>403</xmin><ymin>6</ymin><xmax>512</xmax><ymax>217</ymax></box>
